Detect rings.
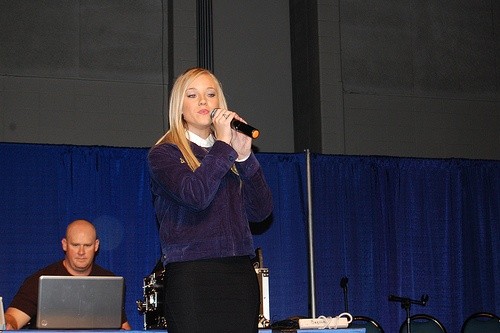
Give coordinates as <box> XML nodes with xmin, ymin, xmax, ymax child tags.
<box><xmin>223</xmin><ymin>114</ymin><xmax>228</xmax><ymax>118</ymax></box>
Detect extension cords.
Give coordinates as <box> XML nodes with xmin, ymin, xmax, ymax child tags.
<box><xmin>299</xmin><ymin>317</ymin><xmax>348</xmax><ymax>329</ymax></box>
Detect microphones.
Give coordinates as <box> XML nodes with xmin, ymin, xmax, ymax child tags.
<box><xmin>211</xmin><ymin>108</ymin><xmax>259</xmax><ymax>139</ymax></box>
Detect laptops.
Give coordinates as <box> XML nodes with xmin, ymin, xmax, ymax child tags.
<box><xmin>36</xmin><ymin>276</ymin><xmax>124</xmax><ymax>330</ymax></box>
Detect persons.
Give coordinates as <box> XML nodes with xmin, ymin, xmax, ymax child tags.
<box><xmin>5</xmin><ymin>220</ymin><xmax>131</xmax><ymax>330</ymax></box>
<box><xmin>148</xmin><ymin>68</ymin><xmax>273</xmax><ymax>333</ymax></box>
